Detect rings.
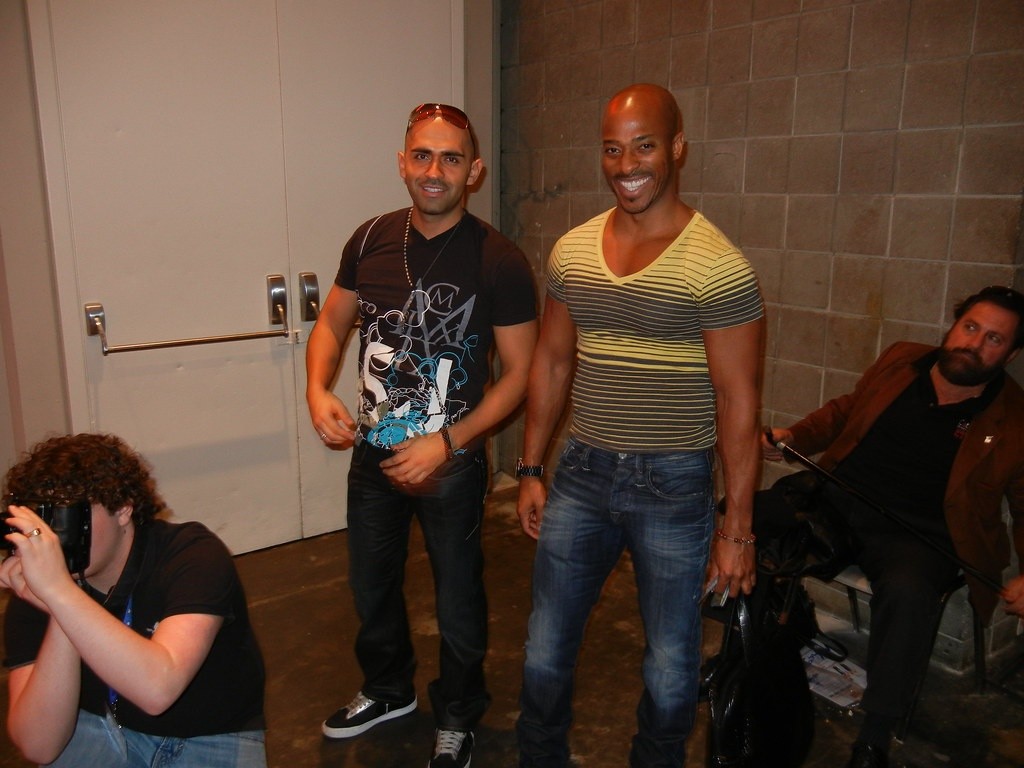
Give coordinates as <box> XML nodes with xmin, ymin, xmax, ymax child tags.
<box><xmin>320</xmin><ymin>434</ymin><xmax>327</xmax><ymax>440</ymax></box>
<box><xmin>26</xmin><ymin>528</ymin><xmax>40</xmax><ymax>538</ymax></box>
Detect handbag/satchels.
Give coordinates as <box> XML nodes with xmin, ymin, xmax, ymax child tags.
<box><xmin>698</xmin><ymin>591</ymin><xmax>849</xmax><ymax>767</ymax></box>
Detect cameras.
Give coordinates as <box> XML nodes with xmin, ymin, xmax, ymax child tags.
<box><xmin>0</xmin><ymin>494</ymin><xmax>93</xmax><ymax>573</ymax></box>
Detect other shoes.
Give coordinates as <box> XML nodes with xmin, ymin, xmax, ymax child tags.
<box><xmin>848</xmin><ymin>720</ymin><xmax>893</xmax><ymax>766</ymax></box>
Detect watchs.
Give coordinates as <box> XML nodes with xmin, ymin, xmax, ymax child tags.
<box><xmin>514</xmin><ymin>456</ymin><xmax>544</xmax><ymax>478</ymax></box>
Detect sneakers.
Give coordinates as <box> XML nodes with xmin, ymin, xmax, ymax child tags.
<box><xmin>426</xmin><ymin>728</ymin><xmax>475</xmax><ymax>768</ymax></box>
<box><xmin>323</xmin><ymin>689</ymin><xmax>417</xmax><ymax>738</ymax></box>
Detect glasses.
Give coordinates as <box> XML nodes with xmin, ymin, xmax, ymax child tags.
<box><xmin>405</xmin><ymin>103</ymin><xmax>477</xmax><ymax>160</ymax></box>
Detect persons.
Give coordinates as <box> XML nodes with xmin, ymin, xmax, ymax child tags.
<box><xmin>513</xmin><ymin>83</ymin><xmax>765</xmax><ymax>768</ymax></box>
<box><xmin>306</xmin><ymin>103</ymin><xmax>540</xmax><ymax>767</ymax></box>
<box><xmin>0</xmin><ymin>433</ymin><xmax>269</xmax><ymax>768</ymax></box>
<box><xmin>701</xmin><ymin>285</ymin><xmax>1024</xmax><ymax>768</ymax></box>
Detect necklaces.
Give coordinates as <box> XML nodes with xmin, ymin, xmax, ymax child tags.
<box><xmin>400</xmin><ymin>207</ymin><xmax>467</xmax><ymax>332</ymax></box>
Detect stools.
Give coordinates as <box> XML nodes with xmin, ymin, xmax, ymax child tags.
<box><xmin>834</xmin><ymin>566</ymin><xmax>988</xmax><ymax>694</ymax></box>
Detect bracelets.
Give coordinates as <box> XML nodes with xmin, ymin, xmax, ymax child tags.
<box><xmin>440</xmin><ymin>428</ymin><xmax>456</xmax><ymax>462</ymax></box>
<box><xmin>717</xmin><ymin>531</ymin><xmax>757</xmax><ymax>545</ymax></box>
